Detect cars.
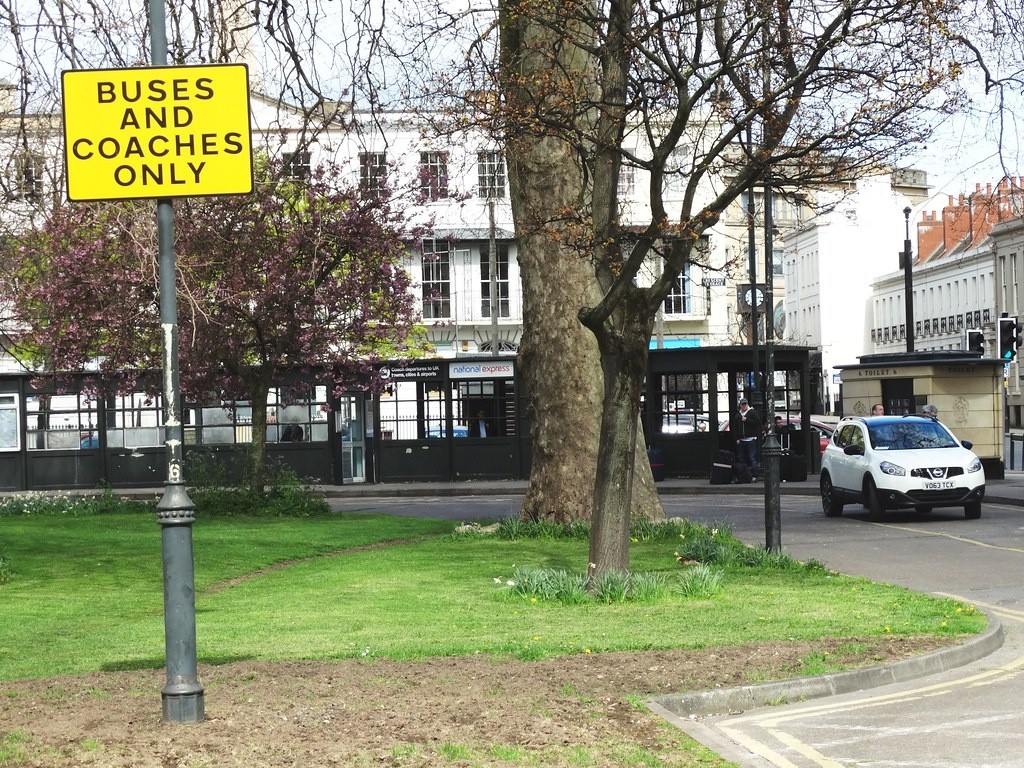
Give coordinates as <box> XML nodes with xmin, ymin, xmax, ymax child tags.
<box><xmin>65</xmin><ymin>436</ymin><xmax>123</xmax><ymax>448</ymax></box>
<box><xmin>662</xmin><ymin>415</ymin><xmax>725</xmax><ymax>432</ymax></box>
<box><xmin>717</xmin><ymin>418</ymin><xmax>834</xmax><ymax>453</ymax></box>
<box><xmin>423</xmin><ymin>426</ymin><xmax>468</xmax><ymax>437</ymax></box>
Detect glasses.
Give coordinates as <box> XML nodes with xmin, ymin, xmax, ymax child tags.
<box><xmin>741</xmin><ymin>403</ymin><xmax>747</xmax><ymax>405</ymax></box>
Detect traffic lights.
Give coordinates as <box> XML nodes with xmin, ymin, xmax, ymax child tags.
<box><xmin>997</xmin><ymin>318</ymin><xmax>1017</xmax><ymax>363</ymax></box>
<box><xmin>967</xmin><ymin>330</ymin><xmax>985</xmax><ymax>359</ymax></box>
<box><xmin>1011</xmin><ymin>316</ymin><xmax>1022</xmax><ymax>349</ymax></box>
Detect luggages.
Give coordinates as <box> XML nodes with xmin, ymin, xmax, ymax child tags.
<box><xmin>779</xmin><ymin>431</ymin><xmax>796</xmax><ymax>482</ymax></box>
<box><xmin>646</xmin><ymin>442</ymin><xmax>665</xmax><ymax>481</ymax></box>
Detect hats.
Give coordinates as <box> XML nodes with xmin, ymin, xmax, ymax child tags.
<box><xmin>740</xmin><ymin>398</ymin><xmax>748</xmax><ymax>404</ymax></box>
<box><xmin>922</xmin><ymin>405</ymin><xmax>938</xmax><ymax>416</ymax></box>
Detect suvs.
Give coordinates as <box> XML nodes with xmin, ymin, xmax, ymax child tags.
<box><xmin>819</xmin><ymin>415</ymin><xmax>985</xmax><ymax>520</ymax></box>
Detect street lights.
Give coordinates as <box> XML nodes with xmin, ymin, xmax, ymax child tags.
<box><xmin>903</xmin><ymin>207</ymin><xmax>915</xmax><ymax>353</ymax></box>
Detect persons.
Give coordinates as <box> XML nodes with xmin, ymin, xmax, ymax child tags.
<box><xmin>732</xmin><ymin>398</ymin><xmax>761</xmax><ymax>482</ymax></box>
<box><xmin>280</xmin><ymin>416</ymin><xmax>303</xmax><ymax>442</ymax></box>
<box><xmin>872</xmin><ymin>404</ymin><xmax>884</xmax><ymax>416</ymax></box>
<box><xmin>470</xmin><ymin>409</ymin><xmax>492</xmax><ymax>437</ymax></box>
<box><xmin>922</xmin><ymin>405</ymin><xmax>937</xmax><ymax>419</ymax></box>
<box><xmin>775</xmin><ymin>416</ymin><xmax>786</xmax><ymax>434</ymax></box>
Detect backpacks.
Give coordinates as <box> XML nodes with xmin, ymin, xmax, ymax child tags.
<box><xmin>709</xmin><ymin>449</ymin><xmax>752</xmax><ymax>485</ymax></box>
